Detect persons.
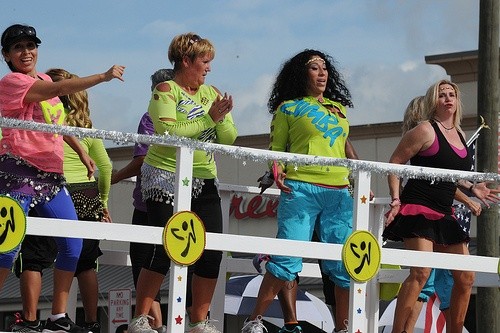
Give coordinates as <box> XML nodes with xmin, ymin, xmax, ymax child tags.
<box><xmin>0</xmin><ymin>24</ymin><xmax>500</xmax><ymax>333</ymax></box>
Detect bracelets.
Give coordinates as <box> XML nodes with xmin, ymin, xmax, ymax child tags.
<box><xmin>468</xmin><ymin>184</ymin><xmax>473</xmax><ymax>191</ymax></box>
<box><xmin>391</xmin><ymin>198</ymin><xmax>401</xmax><ymax>206</ymax></box>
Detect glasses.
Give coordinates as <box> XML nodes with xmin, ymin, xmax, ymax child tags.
<box><xmin>185</xmin><ymin>35</ymin><xmax>201</xmax><ymax>53</ymax></box>
<box><xmin>3</xmin><ymin>26</ymin><xmax>36</xmax><ymax>38</ymax></box>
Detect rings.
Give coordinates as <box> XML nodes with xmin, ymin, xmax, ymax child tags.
<box><xmin>219</xmin><ymin>109</ymin><xmax>222</xmax><ymax>113</ymax></box>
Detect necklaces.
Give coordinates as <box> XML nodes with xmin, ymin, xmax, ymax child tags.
<box><xmin>433</xmin><ymin>118</ymin><xmax>454</xmax><ymax>130</ymax></box>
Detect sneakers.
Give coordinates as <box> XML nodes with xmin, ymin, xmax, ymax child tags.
<box><xmin>76</xmin><ymin>320</ymin><xmax>100</xmax><ymax>333</ymax></box>
<box><xmin>240</xmin><ymin>314</ymin><xmax>268</xmax><ymax>333</ymax></box>
<box><xmin>42</xmin><ymin>312</ymin><xmax>93</xmax><ymax>333</ymax></box>
<box><xmin>128</xmin><ymin>313</ymin><xmax>158</xmax><ymax>333</ymax></box>
<box><xmin>7</xmin><ymin>312</ymin><xmax>43</xmax><ymax>333</ymax></box>
<box><xmin>154</xmin><ymin>324</ymin><xmax>167</xmax><ymax>333</ymax></box>
<box><xmin>189</xmin><ymin>315</ymin><xmax>222</xmax><ymax>333</ymax></box>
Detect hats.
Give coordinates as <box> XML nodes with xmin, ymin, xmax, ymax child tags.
<box><xmin>1</xmin><ymin>24</ymin><xmax>41</xmax><ymax>48</ymax></box>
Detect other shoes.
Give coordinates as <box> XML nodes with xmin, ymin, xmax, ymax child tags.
<box><xmin>279</xmin><ymin>322</ymin><xmax>303</xmax><ymax>333</ymax></box>
